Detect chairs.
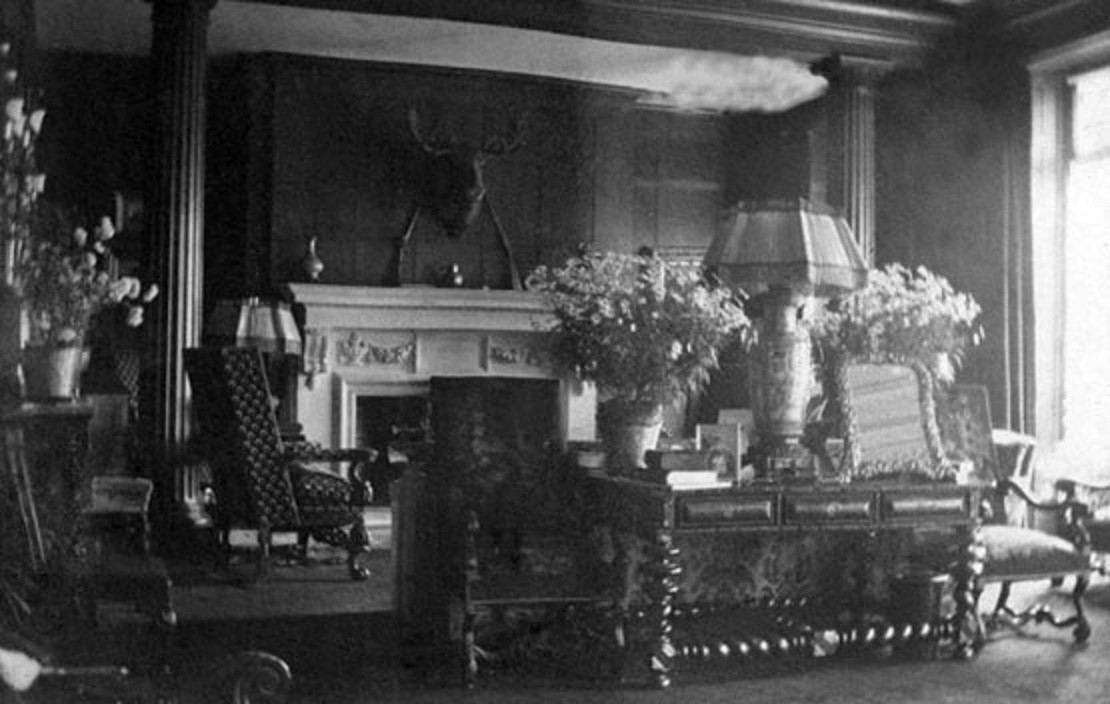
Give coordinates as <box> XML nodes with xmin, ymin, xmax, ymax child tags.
<box><xmin>389</xmin><ymin>374</ymin><xmax>635</xmax><ymax>694</ymax></box>
<box><xmin>185</xmin><ymin>349</ymin><xmax>378</xmax><ymax>578</ymax></box>
<box><xmin>831</xmin><ymin>354</ymin><xmax>1095</xmax><ymax>654</ymax></box>
<box><xmin>0</xmin><ymin>552</ymin><xmax>295</xmax><ymax>704</ymax></box>
<box><xmin>87</xmin><ymin>395</ymin><xmax>151</xmax><ymax>550</ymax></box>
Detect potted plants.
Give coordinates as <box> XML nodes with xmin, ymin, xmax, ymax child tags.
<box><xmin>492</xmin><ymin>247</ymin><xmax>759</xmax><ymax>465</ymax></box>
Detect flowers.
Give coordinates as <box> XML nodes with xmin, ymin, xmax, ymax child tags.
<box><xmin>0</xmin><ymin>42</ymin><xmax>160</xmax><ymax>343</ymax></box>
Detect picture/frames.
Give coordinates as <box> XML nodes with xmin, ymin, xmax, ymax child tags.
<box><xmin>697</xmin><ymin>425</ymin><xmax>742</xmax><ymax>483</ymax></box>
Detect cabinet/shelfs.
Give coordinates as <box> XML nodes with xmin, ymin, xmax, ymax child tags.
<box><xmin>574</xmin><ymin>461</ymin><xmax>979</xmax><ymax>691</ymax></box>
<box><xmin>0</xmin><ymin>401</ymin><xmax>95</xmax><ymax>581</ymax></box>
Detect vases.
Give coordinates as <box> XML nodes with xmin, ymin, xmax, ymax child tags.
<box><xmin>24</xmin><ymin>327</ymin><xmax>84</xmax><ymax>399</ymax></box>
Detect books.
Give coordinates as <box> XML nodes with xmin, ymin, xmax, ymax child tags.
<box><xmin>645</xmin><ymin>447</ymin><xmax>734</xmax><ymax>493</ymax></box>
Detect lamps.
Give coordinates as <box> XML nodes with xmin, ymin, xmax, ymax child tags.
<box><xmin>209</xmin><ymin>299</ymin><xmax>303</xmax><ymax>415</ymax></box>
<box><xmin>701</xmin><ymin>196</ymin><xmax>871</xmax><ymax>473</ymax></box>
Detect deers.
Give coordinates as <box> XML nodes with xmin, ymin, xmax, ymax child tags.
<box><xmin>416</xmin><ymin>105</ymin><xmax>527</xmax><ymax>226</ymax></box>
